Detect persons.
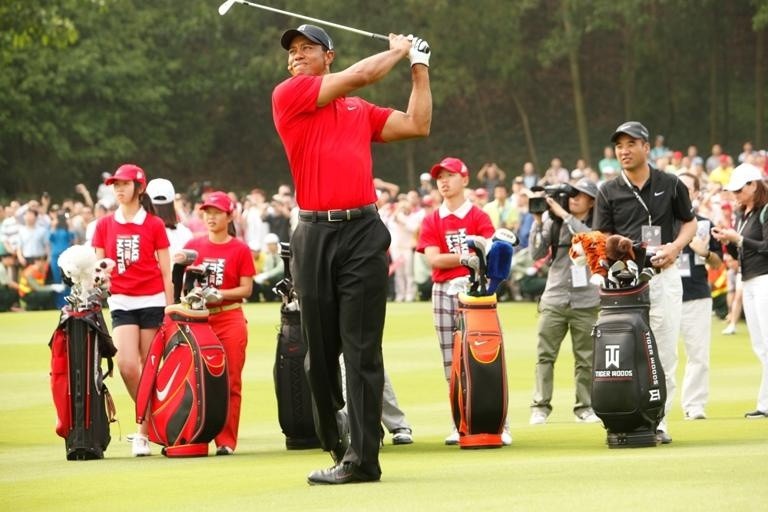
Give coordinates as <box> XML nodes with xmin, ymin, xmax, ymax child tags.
<box><xmin>271</xmin><ymin>25</ymin><xmax>433</xmax><ymax>486</ymax></box>
<box><xmin>527</xmin><ymin>178</ymin><xmax>603</xmax><ymax>422</ymax></box>
<box><xmin>376</xmin><ymin>370</ymin><xmax>415</xmax><ymax>444</ymax></box>
<box><xmin>412</xmin><ymin>157</ymin><xmax>519</xmax><ymax>445</ymax></box>
<box><xmin>176</xmin><ymin>188</ymin><xmax>256</xmax><ymax>455</ymax></box>
<box><xmin>0</xmin><ymin>172</ymin><xmax>300</xmax><ymax>310</ymax></box>
<box><xmin>375</xmin><ymin>134</ymin><xmax>768</xmax><ymax>332</ymax></box>
<box><xmin>669</xmin><ymin>171</ymin><xmax>723</xmax><ymax>419</ymax></box>
<box><xmin>93</xmin><ymin>164</ymin><xmax>176</xmax><ymax>456</ymax></box>
<box><xmin>709</xmin><ymin>162</ymin><xmax>768</xmax><ymax>418</ymax></box>
<box><xmin>593</xmin><ymin>121</ymin><xmax>699</xmax><ymax>444</ymax></box>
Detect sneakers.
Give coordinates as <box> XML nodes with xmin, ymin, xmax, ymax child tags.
<box><xmin>722</xmin><ymin>325</ymin><xmax>736</xmax><ymax>335</ymax></box>
<box><xmin>216</xmin><ymin>444</ymin><xmax>235</xmax><ymax>456</ymax></box>
<box><xmin>126</xmin><ymin>431</ymin><xmax>152</xmax><ymax>458</ymax></box>
<box><xmin>576</xmin><ymin>410</ymin><xmax>602</xmax><ymax>425</ymax></box>
<box><xmin>529</xmin><ymin>409</ymin><xmax>548</xmax><ymax>426</ymax></box>
<box><xmin>744</xmin><ymin>408</ymin><xmax>768</xmax><ymax>419</ymax></box>
<box><xmin>445</xmin><ymin>428</ymin><xmax>513</xmax><ymax>446</ymax></box>
<box><xmin>649</xmin><ymin>429</ymin><xmax>673</xmax><ymax>444</ymax></box>
<box><xmin>392</xmin><ymin>426</ymin><xmax>413</xmax><ymax>446</ymax></box>
<box><xmin>307</xmin><ymin>457</ymin><xmax>382</xmax><ymax>487</ymax></box>
<box><xmin>683</xmin><ymin>411</ymin><xmax>707</xmax><ymax>420</ymax></box>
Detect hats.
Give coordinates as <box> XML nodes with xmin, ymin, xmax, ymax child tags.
<box><xmin>144</xmin><ymin>178</ymin><xmax>177</xmax><ymax>205</ymax></box>
<box><xmin>104</xmin><ymin>163</ymin><xmax>146</xmax><ymax>187</ymax></box>
<box><xmin>431</xmin><ymin>157</ymin><xmax>468</xmax><ymax>180</ymax></box>
<box><xmin>722</xmin><ymin>163</ymin><xmax>763</xmax><ymax>193</ymax></box>
<box><xmin>610</xmin><ymin>121</ymin><xmax>650</xmax><ymax>143</ymax></box>
<box><xmin>281</xmin><ymin>23</ymin><xmax>335</xmax><ymax>51</ymax></box>
<box><xmin>199</xmin><ymin>190</ymin><xmax>235</xmax><ymax>214</ymax></box>
<box><xmin>565</xmin><ymin>177</ymin><xmax>599</xmax><ymax>199</ymax></box>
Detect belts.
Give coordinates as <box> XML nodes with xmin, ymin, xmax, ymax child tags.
<box><xmin>207</xmin><ymin>302</ymin><xmax>242</xmax><ymax>315</ymax></box>
<box><xmin>298</xmin><ymin>202</ymin><xmax>379</xmax><ymax>224</ymax></box>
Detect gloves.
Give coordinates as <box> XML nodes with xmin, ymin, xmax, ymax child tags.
<box><xmin>403</xmin><ymin>34</ymin><xmax>431</xmax><ymax>68</ymax></box>
<box><xmin>459</xmin><ymin>254</ymin><xmax>482</xmax><ymax>273</ymax></box>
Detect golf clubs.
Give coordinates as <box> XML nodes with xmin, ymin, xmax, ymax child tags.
<box><xmin>272</xmin><ymin>278</ymin><xmax>298</xmax><ymax>310</ymax></box>
<box><xmin>590</xmin><ymin>261</ymin><xmax>654</xmax><ymax>290</ymax></box>
<box><xmin>63</xmin><ymin>286</ymin><xmax>103</xmax><ymax>312</ymax></box>
<box><xmin>172</xmin><ymin>249</ymin><xmax>222</xmax><ymax>311</ymax></box>
<box><xmin>218</xmin><ymin>0</ymin><xmax>429</xmax><ymax>56</ymax></box>
<box><xmin>459</xmin><ymin>228</ymin><xmax>520</xmax><ymax>294</ymax></box>
